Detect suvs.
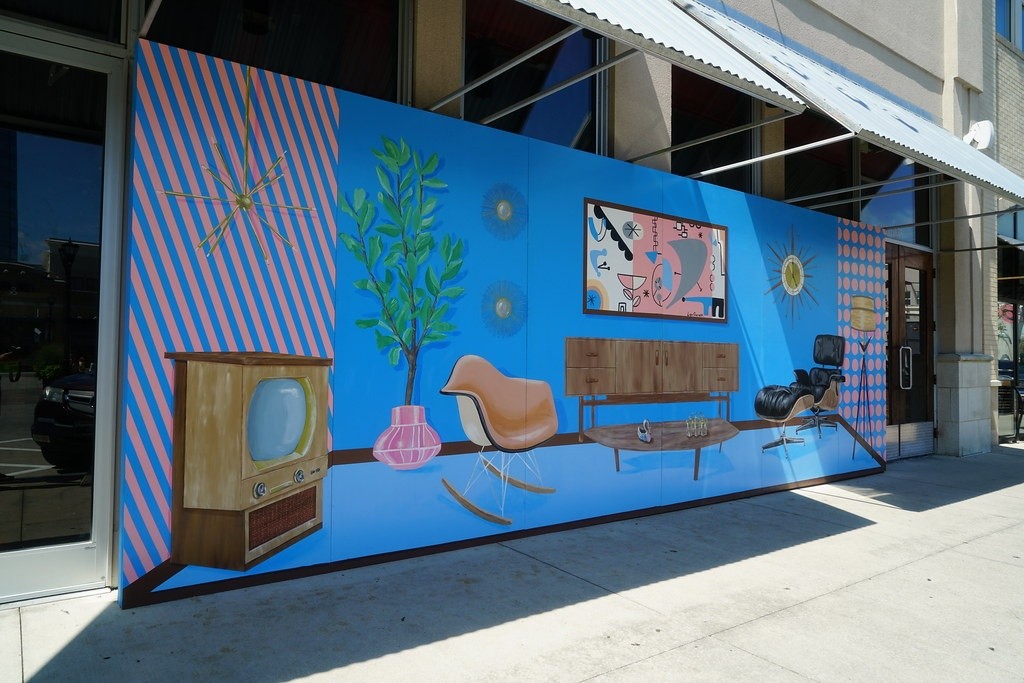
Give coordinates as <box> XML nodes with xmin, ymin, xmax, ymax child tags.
<box><xmin>29</xmin><ymin>360</ymin><xmax>98</xmax><ymax>471</ymax></box>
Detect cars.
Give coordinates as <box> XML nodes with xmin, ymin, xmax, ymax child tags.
<box><xmin>0</xmin><ymin>260</ymin><xmax>98</xmax><ymax>338</ymax></box>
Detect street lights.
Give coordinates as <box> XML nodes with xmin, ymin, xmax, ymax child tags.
<box><xmin>57</xmin><ymin>237</ymin><xmax>80</xmax><ymax>366</ymax></box>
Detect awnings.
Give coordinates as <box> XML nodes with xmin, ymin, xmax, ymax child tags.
<box><xmin>674</xmin><ymin>1</ymin><xmax>1023</xmax><ymax>251</ymax></box>
<box><xmin>419</xmin><ymin>1</ymin><xmax>809</xmax><ymax>162</ymax></box>
